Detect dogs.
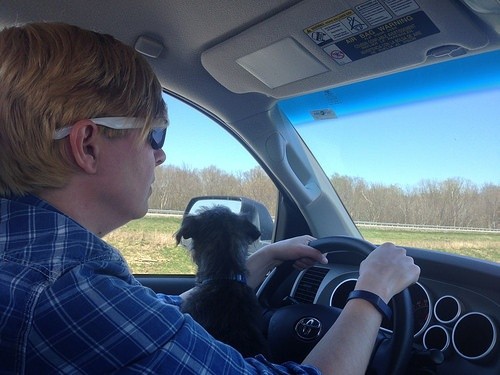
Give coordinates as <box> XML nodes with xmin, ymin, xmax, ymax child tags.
<box><xmin>172</xmin><ymin>203</ymin><xmax>273</xmax><ymax>362</ymax></box>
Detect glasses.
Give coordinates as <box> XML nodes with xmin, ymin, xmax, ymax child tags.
<box><xmin>52</xmin><ymin>117</ymin><xmax>168</xmax><ymax>150</ymax></box>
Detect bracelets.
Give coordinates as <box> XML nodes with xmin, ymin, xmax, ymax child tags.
<box><xmin>347</xmin><ymin>290</ymin><xmax>392</xmax><ymax>321</ymax></box>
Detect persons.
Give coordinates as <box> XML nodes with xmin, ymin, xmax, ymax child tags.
<box><xmin>0</xmin><ymin>21</ymin><xmax>421</xmax><ymax>375</ymax></box>
